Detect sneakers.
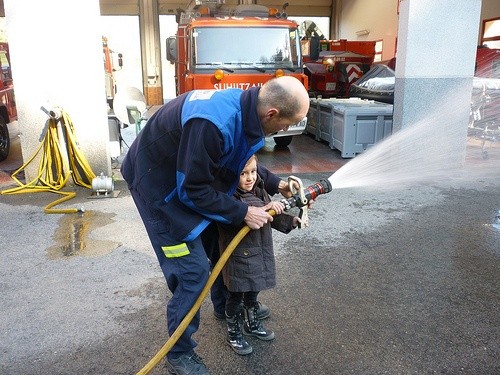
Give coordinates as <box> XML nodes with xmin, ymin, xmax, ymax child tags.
<box><xmin>169</xmin><ymin>350</ymin><xmax>209</xmax><ymax>375</ymax></box>
<box><xmin>214</xmin><ymin>303</ymin><xmax>270</xmax><ymax>321</ymax></box>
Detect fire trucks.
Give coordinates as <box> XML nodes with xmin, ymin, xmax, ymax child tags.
<box><xmin>166</xmin><ymin>0</ymin><xmax>309</xmax><ymax>145</ymax></box>
<box><xmin>0</xmin><ymin>42</ymin><xmax>19</xmax><ymax>162</ymax></box>
<box><xmin>102</xmin><ymin>35</ymin><xmax>123</xmax><ymax>108</ymax></box>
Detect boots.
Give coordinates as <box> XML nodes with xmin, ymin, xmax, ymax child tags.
<box><xmin>225</xmin><ymin>311</ymin><xmax>252</xmax><ymax>354</ymax></box>
<box><xmin>242</xmin><ymin>302</ymin><xmax>274</xmax><ymax>341</ymax></box>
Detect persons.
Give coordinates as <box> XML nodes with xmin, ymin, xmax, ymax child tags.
<box><xmin>120</xmin><ymin>76</ymin><xmax>308</xmax><ymax>374</ymax></box>
<box><xmin>219</xmin><ymin>155</ymin><xmax>310</xmax><ymax>356</ymax></box>
